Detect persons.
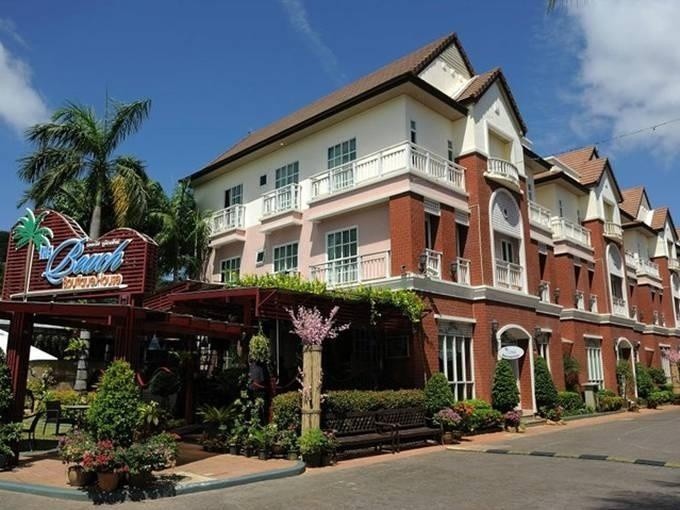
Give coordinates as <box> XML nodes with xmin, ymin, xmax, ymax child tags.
<box><xmin>248</xmin><ymin>375</ymin><xmax>268</xmax><ymax>390</ymax></box>
<box><xmin>41</xmin><ymin>366</ymin><xmax>58</xmax><ymax>388</ymax></box>
<box><xmin>269</xmin><ymin>375</ymin><xmax>280</xmax><ymax>387</ymax></box>
<box><xmin>286</xmin><ymin>364</ymin><xmax>302</xmax><ymax>391</ymax></box>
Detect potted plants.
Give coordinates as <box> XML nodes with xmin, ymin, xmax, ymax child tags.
<box><xmin>228</xmin><ymin>427</ymin><xmax>341</xmax><ymax>468</ymax></box>
<box><xmin>58</xmin><ymin>429</ymin><xmax>181</xmax><ymax>492</ymax></box>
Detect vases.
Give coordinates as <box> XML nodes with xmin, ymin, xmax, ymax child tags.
<box><xmin>442</xmin><ymin>431</ymin><xmax>455</xmax><ymax>444</ymax></box>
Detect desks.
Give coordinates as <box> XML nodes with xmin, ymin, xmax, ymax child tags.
<box><xmin>64</xmin><ymin>405</ymin><xmax>90</xmax><ymax>430</ymax></box>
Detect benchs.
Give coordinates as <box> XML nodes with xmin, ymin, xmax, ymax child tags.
<box><xmin>321</xmin><ymin>407</ymin><xmax>444</xmax><ymax>463</ymax></box>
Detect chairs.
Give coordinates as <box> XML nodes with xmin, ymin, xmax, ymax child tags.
<box><xmin>18</xmin><ymin>411</ymin><xmax>45</xmax><ymax>451</ymax></box>
<box><xmin>42</xmin><ymin>400</ymin><xmax>75</xmax><ymax>436</ymax></box>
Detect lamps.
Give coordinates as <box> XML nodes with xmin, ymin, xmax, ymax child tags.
<box><xmin>419</xmin><ymin>253</ymin><xmax>595</xmax><ymax>337</ymax></box>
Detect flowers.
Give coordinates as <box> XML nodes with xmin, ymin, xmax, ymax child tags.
<box><xmin>434</xmin><ymin>409</ymin><xmax>463</xmax><ymax>432</ymax></box>
